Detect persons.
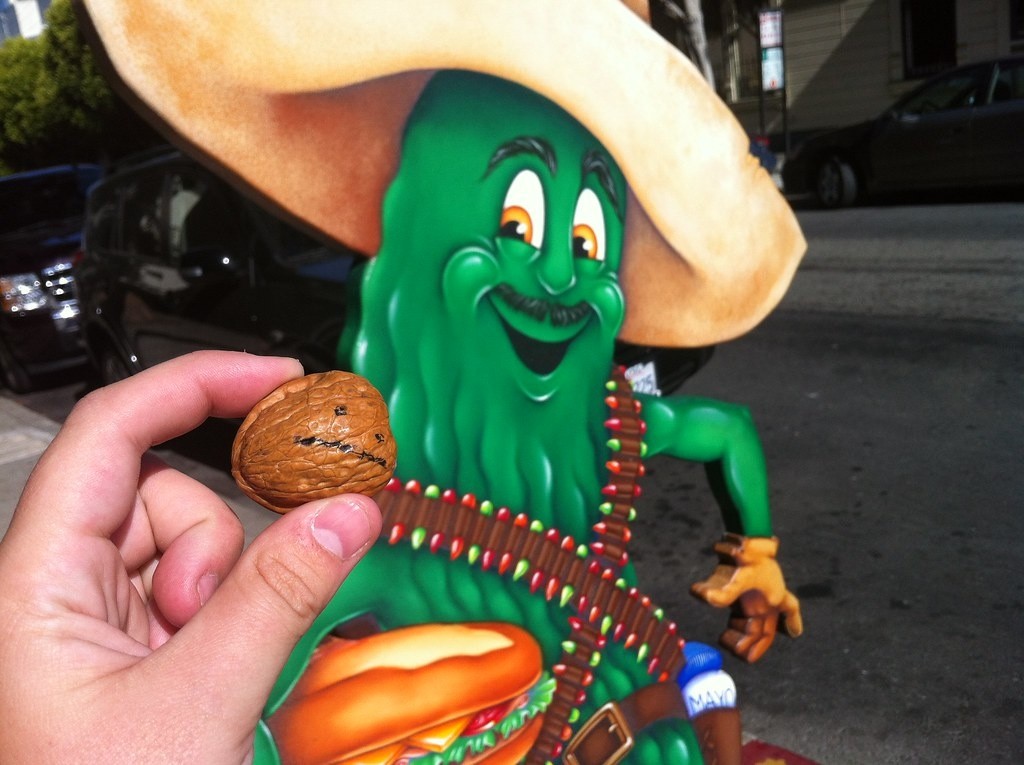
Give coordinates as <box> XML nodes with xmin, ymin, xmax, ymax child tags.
<box><xmin>0</xmin><ymin>349</ymin><xmax>382</xmax><ymax>765</ymax></box>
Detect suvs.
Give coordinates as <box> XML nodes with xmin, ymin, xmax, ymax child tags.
<box><xmin>73</xmin><ymin>144</ymin><xmax>714</xmax><ymax>474</ymax></box>
<box><xmin>0</xmin><ymin>162</ymin><xmax>109</xmax><ymax>396</ymax></box>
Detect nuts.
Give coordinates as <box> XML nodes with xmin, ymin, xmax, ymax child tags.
<box><xmin>232</xmin><ymin>370</ymin><xmax>397</xmax><ymax>514</ymax></box>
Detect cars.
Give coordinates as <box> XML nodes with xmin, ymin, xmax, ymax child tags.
<box><xmin>792</xmin><ymin>56</ymin><xmax>1024</xmax><ymax>211</ymax></box>
